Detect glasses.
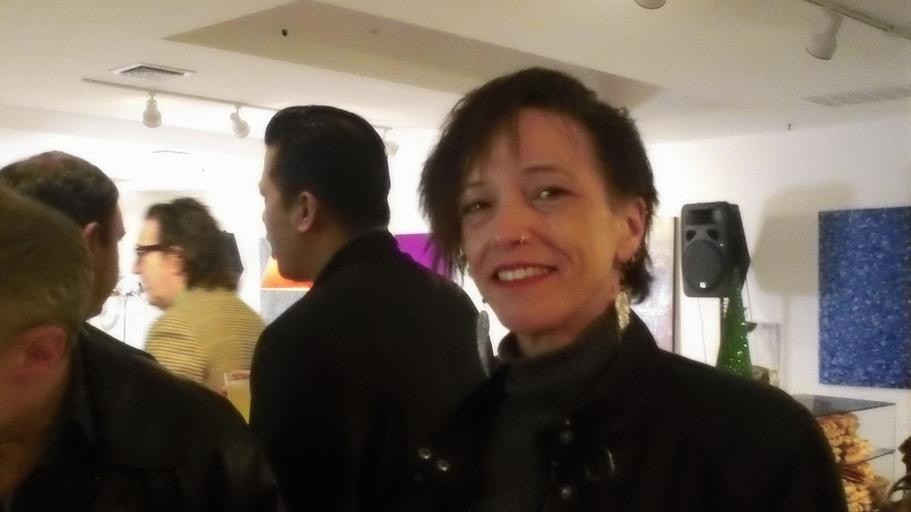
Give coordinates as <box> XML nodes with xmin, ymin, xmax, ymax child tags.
<box><xmin>137</xmin><ymin>242</ymin><xmax>168</xmax><ymax>257</ymax></box>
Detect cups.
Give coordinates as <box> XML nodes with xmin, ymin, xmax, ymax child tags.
<box><xmin>224</xmin><ymin>370</ymin><xmax>251</xmax><ymax>426</ymax></box>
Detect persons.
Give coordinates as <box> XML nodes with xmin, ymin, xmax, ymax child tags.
<box><xmin>417</xmin><ymin>65</ymin><xmax>847</xmax><ymax>510</ymax></box>
<box><xmin>1</xmin><ymin>152</ymin><xmax>157</xmax><ymax>359</ymax></box>
<box><xmin>2</xmin><ymin>189</ymin><xmax>291</xmax><ymax>511</ymax></box>
<box><xmin>132</xmin><ymin>194</ymin><xmax>266</xmax><ymax>424</ymax></box>
<box><xmin>222</xmin><ymin>105</ymin><xmax>495</xmax><ymax>510</ymax></box>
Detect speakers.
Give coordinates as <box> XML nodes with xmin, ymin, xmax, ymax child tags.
<box><xmin>679</xmin><ymin>201</ymin><xmax>750</xmax><ymax>297</ymax></box>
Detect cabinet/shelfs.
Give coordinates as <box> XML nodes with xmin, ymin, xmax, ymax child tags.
<box><xmin>790</xmin><ymin>392</ymin><xmax>895</xmax><ymax>512</ymax></box>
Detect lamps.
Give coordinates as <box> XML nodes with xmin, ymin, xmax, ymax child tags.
<box><xmin>633</xmin><ymin>0</ymin><xmax>911</xmax><ymax>61</ymax></box>
<box><xmin>83</xmin><ymin>77</ymin><xmax>400</xmax><ymax>155</ymax></box>
